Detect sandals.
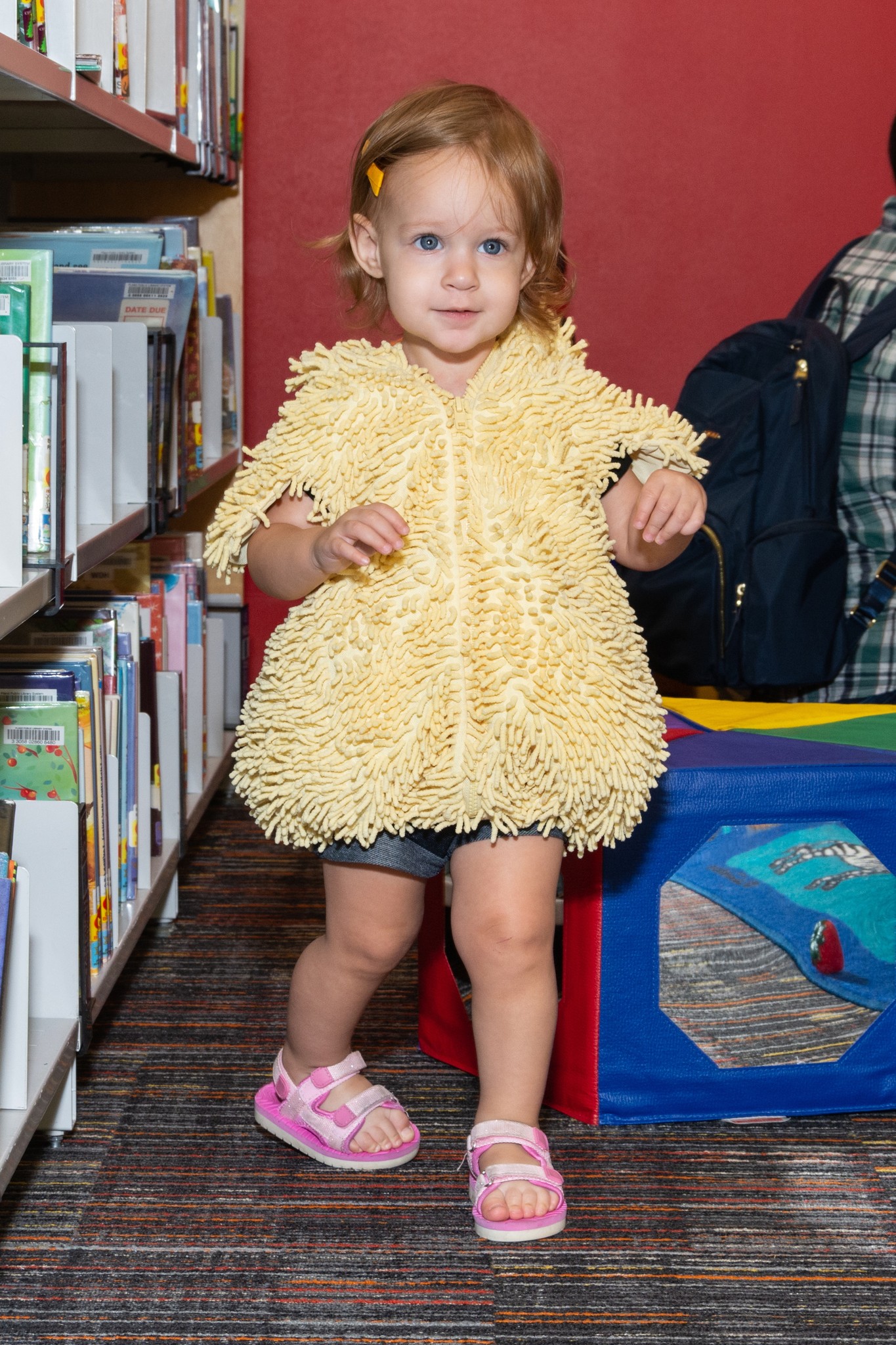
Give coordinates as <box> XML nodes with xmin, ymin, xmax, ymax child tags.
<box><xmin>254</xmin><ymin>1046</ymin><xmax>421</xmax><ymax>1170</ymax></box>
<box><xmin>465</xmin><ymin>1118</ymin><xmax>568</xmax><ymax>1242</ymax></box>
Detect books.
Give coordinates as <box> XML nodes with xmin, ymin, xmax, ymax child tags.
<box><xmin>0</xmin><ymin>0</ymin><xmax>244</xmax><ymax>162</ymax></box>
<box><xmin>0</xmin><ymin>216</ymin><xmax>242</xmax><ymax>1114</ymax></box>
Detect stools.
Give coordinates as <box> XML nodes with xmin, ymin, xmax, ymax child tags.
<box><xmin>414</xmin><ymin>698</ymin><xmax>896</xmax><ymax>1128</ymax></box>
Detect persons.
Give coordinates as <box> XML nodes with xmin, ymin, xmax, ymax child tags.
<box><xmin>789</xmin><ymin>108</ymin><xmax>896</xmax><ymax>716</ymax></box>
<box><xmin>200</xmin><ymin>74</ymin><xmax>712</xmax><ymax>1244</ymax></box>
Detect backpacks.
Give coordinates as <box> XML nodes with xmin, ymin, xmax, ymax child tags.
<box><xmin>613</xmin><ymin>226</ymin><xmax>896</xmax><ymax>699</ymax></box>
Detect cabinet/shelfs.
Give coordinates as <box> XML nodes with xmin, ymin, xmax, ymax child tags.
<box><xmin>0</xmin><ymin>0</ymin><xmax>246</xmax><ymax>1199</ymax></box>
